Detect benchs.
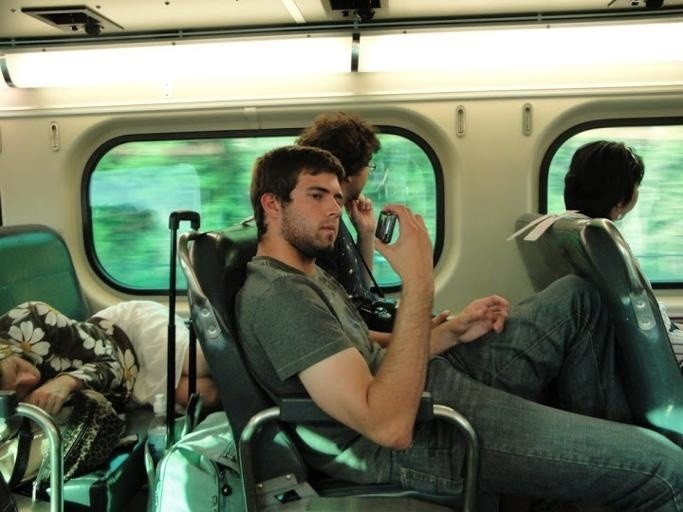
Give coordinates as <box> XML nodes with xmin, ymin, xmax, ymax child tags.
<box><xmin>176</xmin><ymin>219</ymin><xmax>485</xmax><ymax>512</ymax></box>
<box><xmin>2</xmin><ymin>221</ymin><xmax>152</xmax><ymax>512</ymax></box>
<box><xmin>512</xmin><ymin>207</ymin><xmax>682</xmax><ymax>452</ymax></box>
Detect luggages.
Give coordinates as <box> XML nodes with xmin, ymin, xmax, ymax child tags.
<box><xmin>143</xmin><ymin>210</ymin><xmax>245</xmax><ymax>512</ymax></box>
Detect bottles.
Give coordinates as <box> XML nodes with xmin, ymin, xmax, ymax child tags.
<box><xmin>147</xmin><ymin>392</ymin><xmax>177</xmax><ymax>469</ymax></box>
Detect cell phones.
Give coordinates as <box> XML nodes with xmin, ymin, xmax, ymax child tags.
<box><xmin>375</xmin><ymin>211</ymin><xmax>397</xmax><ymax>243</ymax></box>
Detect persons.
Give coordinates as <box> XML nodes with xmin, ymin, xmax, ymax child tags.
<box><xmin>231</xmin><ymin>145</ymin><xmax>683</xmax><ymax>512</ymax></box>
<box><xmin>563</xmin><ymin>141</ymin><xmax>683</xmax><ymax>374</ymax></box>
<box><xmin>1</xmin><ymin>299</ymin><xmax>221</xmax><ymax>416</ymax></box>
<box><xmin>291</xmin><ymin>111</ymin><xmax>450</xmax><ymax>348</ymax></box>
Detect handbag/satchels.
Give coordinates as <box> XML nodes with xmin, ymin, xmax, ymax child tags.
<box><xmin>31</xmin><ymin>389</ymin><xmax>122</xmax><ymax>505</ymax></box>
